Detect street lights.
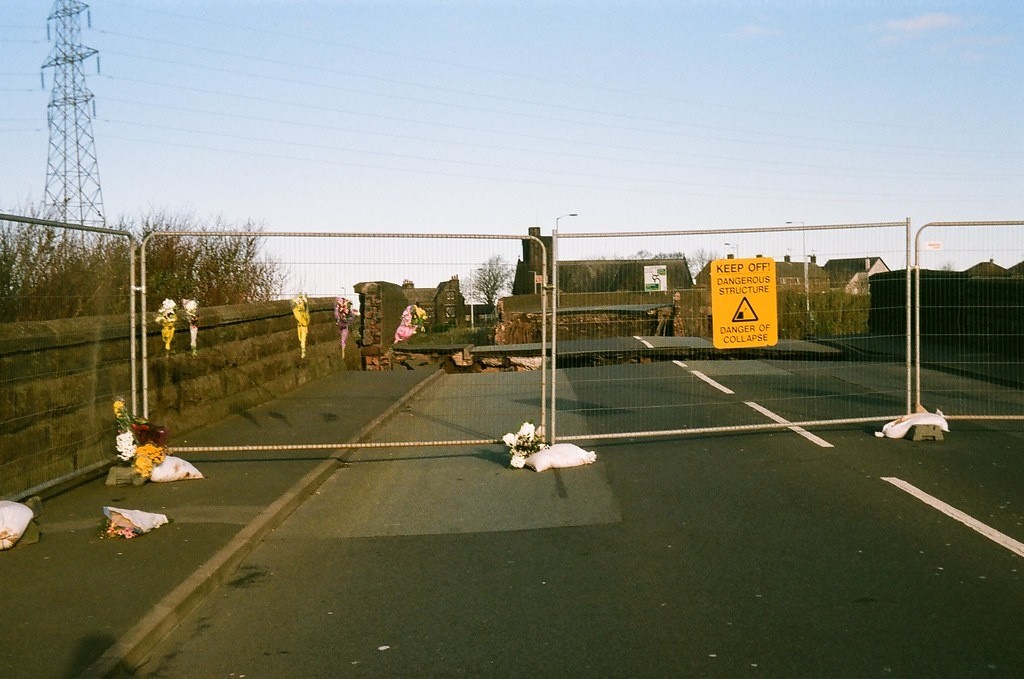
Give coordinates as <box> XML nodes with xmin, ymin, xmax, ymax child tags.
<box><xmin>724</xmin><ymin>242</ymin><xmax>739</xmax><ymax>258</ymax></box>
<box><xmin>469</xmin><ymin>268</ymin><xmax>483</xmax><ymax>328</ymax></box>
<box><xmin>341</xmin><ymin>286</ymin><xmax>346</xmax><ymax>298</ymax></box>
<box><xmin>528</xmin><ymin>271</ymin><xmax>536</xmax><ymax>293</ymax></box>
<box><xmin>785</xmin><ymin>221</ymin><xmax>809</xmax><ymax>310</ymax></box>
<box><xmin>555</xmin><ymin>212</ymin><xmax>579</xmax><ymax>309</ymax></box>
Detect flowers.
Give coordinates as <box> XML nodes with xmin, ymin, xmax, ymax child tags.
<box><xmin>109</xmin><ymin>396</ymin><xmax>169</xmax><ymax>477</ymax></box>
<box><xmin>289</xmin><ymin>291</ymin><xmax>310</xmax><ymax>358</ymax></box>
<box><xmin>182</xmin><ymin>297</ymin><xmax>201</xmax><ymax>358</ymax></box>
<box><xmin>393</xmin><ymin>302</ymin><xmax>429</xmax><ymax>343</ymax></box>
<box><xmin>155</xmin><ymin>298</ymin><xmax>179</xmax><ymax>359</ymax></box>
<box><xmin>501</xmin><ymin>421</ymin><xmax>551</xmax><ymax>469</ymax></box>
<box><xmin>97</xmin><ymin>505</ymin><xmax>175</xmax><ymax>539</ymax></box>
<box><xmin>333</xmin><ymin>297</ymin><xmax>356</xmax><ymax>359</ymax></box>
